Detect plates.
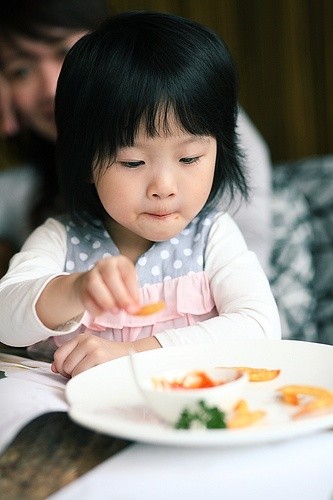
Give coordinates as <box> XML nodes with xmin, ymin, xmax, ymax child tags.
<box><xmin>64</xmin><ymin>339</ymin><xmax>333</xmax><ymax>448</ymax></box>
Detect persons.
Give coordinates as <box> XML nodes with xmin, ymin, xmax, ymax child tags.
<box><xmin>0</xmin><ymin>13</ymin><xmax>281</xmax><ymax>377</ymax></box>
<box><xmin>0</xmin><ymin>0</ymin><xmax>272</xmax><ymax>281</ymax></box>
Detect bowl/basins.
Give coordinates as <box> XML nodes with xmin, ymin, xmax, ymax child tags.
<box><xmin>128</xmin><ymin>348</ymin><xmax>247</xmax><ymax>426</ymax></box>
